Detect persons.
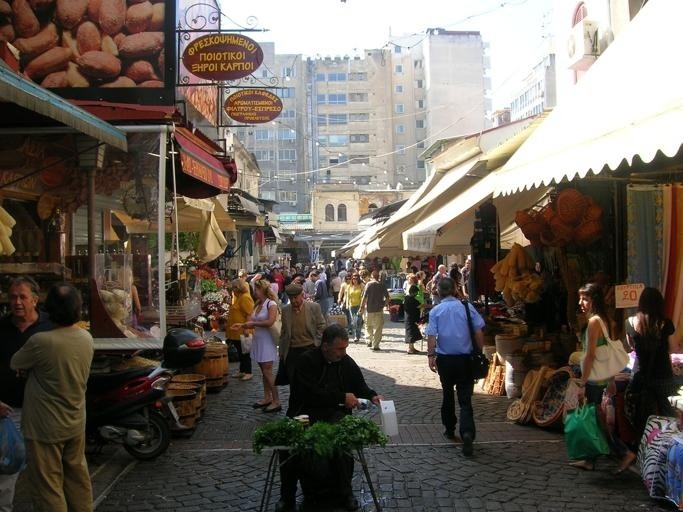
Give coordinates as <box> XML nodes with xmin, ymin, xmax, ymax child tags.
<box><xmin>1</xmin><ymin>274</ymin><xmax>49</xmax><ymax>510</ymax></box>
<box><xmin>272</xmin><ymin>324</ymin><xmax>381</xmax><ymax>510</ymax></box>
<box><xmin>223</xmin><ymin>252</ymin><xmax>474</xmax><ymax>414</ymax></box>
<box><xmin>115</xmin><ymin>260</ymin><xmax>198</xmax><ymax>329</ymax></box>
<box><xmin>619</xmin><ymin>285</ymin><xmax>678</xmax><ymax>465</ymax></box>
<box><xmin>426</xmin><ymin>280</ymin><xmax>486</xmax><ymax>454</ymax></box>
<box><xmin>569</xmin><ymin>284</ymin><xmax>637</xmax><ymax>474</ymax></box>
<box><xmin>7</xmin><ymin>283</ymin><xmax>97</xmax><ymax>512</ymax></box>
<box><xmin>1</xmin><ymin>400</ymin><xmax>14</xmax><ymax>420</ymax></box>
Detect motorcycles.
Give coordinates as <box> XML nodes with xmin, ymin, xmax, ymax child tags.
<box><xmin>85</xmin><ymin>329</ymin><xmax>207</xmax><ymax>461</ymax></box>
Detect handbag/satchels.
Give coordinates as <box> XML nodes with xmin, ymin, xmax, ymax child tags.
<box><xmin>563</xmin><ymin>402</ymin><xmax>609</xmax><ymax>460</ymax></box>
<box><xmin>580</xmin><ymin>316</ymin><xmax>629</xmax><ymax>381</ymax></box>
<box><xmin>470</xmin><ymin>349</ymin><xmax>489</xmax><ymax>378</ymax></box>
<box><xmin>269</xmin><ymin>314</ymin><xmax>282</xmax><ymax>345</ymax></box>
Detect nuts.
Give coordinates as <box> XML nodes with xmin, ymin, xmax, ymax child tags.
<box><xmin>0</xmin><ymin>0</ymin><xmax>166</xmax><ymax>89</ymax></box>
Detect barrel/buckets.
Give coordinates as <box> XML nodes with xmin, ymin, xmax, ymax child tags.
<box><xmin>505</xmin><ymin>353</ymin><xmax>528</xmax><ymax>397</ymax></box>
<box><xmin>166</xmin><ymin>343</ymin><xmax>228</xmax><ymax>432</ymax></box>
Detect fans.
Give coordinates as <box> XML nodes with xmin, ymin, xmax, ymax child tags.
<box><xmin>122</xmin><ymin>170</ymin><xmax>156</xmax><ymax>220</ymax></box>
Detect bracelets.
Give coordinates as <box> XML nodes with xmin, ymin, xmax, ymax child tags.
<box><xmin>578</xmin><ymin>384</ymin><xmax>586</xmax><ymax>388</ymax></box>
<box><xmin>428</xmin><ymin>352</ymin><xmax>435</xmax><ymax>358</ymax></box>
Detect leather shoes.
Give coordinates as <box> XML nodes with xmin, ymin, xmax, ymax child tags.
<box><xmin>463</xmin><ymin>431</ymin><xmax>473</xmax><ymax>457</ymax></box>
<box><xmin>253</xmin><ymin>402</ymin><xmax>281</xmax><ymax>412</ymax></box>
<box><xmin>444</xmin><ymin>430</ymin><xmax>454</xmax><ymax>438</ymax></box>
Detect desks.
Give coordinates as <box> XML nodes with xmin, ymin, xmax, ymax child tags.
<box><xmin>259</xmin><ymin>444</ymin><xmax>382</xmax><ymax>512</ymax></box>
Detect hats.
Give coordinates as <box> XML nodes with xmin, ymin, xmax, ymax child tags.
<box><xmin>286</xmin><ymin>284</ymin><xmax>303</xmax><ymax>295</ymax></box>
